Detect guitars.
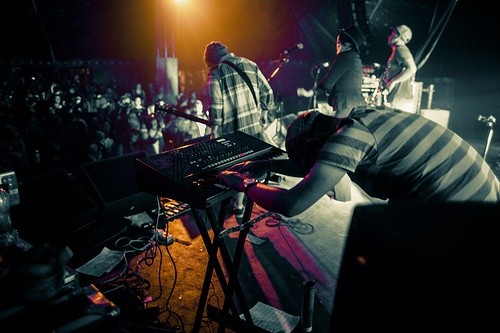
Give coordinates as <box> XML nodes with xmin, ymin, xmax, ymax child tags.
<box><xmin>147</xmin><ymin>101</ymin><xmax>210</xmax><ymax>126</ymax></box>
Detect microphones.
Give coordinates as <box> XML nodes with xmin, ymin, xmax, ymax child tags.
<box><xmin>314</xmin><ymin>62</ymin><xmax>329</xmax><ymax>68</ymax></box>
<box><xmin>284</xmin><ymin>42</ymin><xmax>304</xmax><ymax>54</ymax></box>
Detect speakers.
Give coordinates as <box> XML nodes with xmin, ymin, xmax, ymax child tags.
<box><xmin>81</xmin><ymin>151</ymin><xmax>157</xmax><ymax>217</ymax></box>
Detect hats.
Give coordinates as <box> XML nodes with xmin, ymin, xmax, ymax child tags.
<box><xmin>337</xmin><ymin>24</ymin><xmax>364</xmax><ymax>53</ymax></box>
<box><xmin>285</xmin><ymin>111</ymin><xmax>320</xmax><ymax>172</ymax></box>
<box><xmin>391</xmin><ymin>25</ymin><xmax>413</xmax><ymax>46</ymax></box>
<box><xmin>205</xmin><ymin>42</ymin><xmax>227</xmax><ymax>67</ymax></box>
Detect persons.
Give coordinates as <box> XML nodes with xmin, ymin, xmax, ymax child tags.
<box><xmin>0</xmin><ymin>70</ymin><xmax>213</xmax><ymax>199</ymax></box>
<box><xmin>216</xmin><ymin>105</ymin><xmax>500</xmax><ymax>218</ymax></box>
<box><xmin>297</xmin><ymin>31</ymin><xmax>365</xmax><ymax>202</ymax></box>
<box><xmin>205</xmin><ymin>42</ymin><xmax>274</xmax><ymax>218</ymax></box>
<box><xmin>381</xmin><ymin>26</ymin><xmax>417</xmax><ymax>114</ymax></box>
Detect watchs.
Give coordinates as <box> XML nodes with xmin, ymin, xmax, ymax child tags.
<box><xmin>244</xmin><ymin>178</ymin><xmax>261</xmax><ymax>196</ymax></box>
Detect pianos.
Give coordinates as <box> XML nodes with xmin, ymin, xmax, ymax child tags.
<box><xmin>136</xmin><ymin>129</ymin><xmax>289</xmax><ymax>211</ymax></box>
